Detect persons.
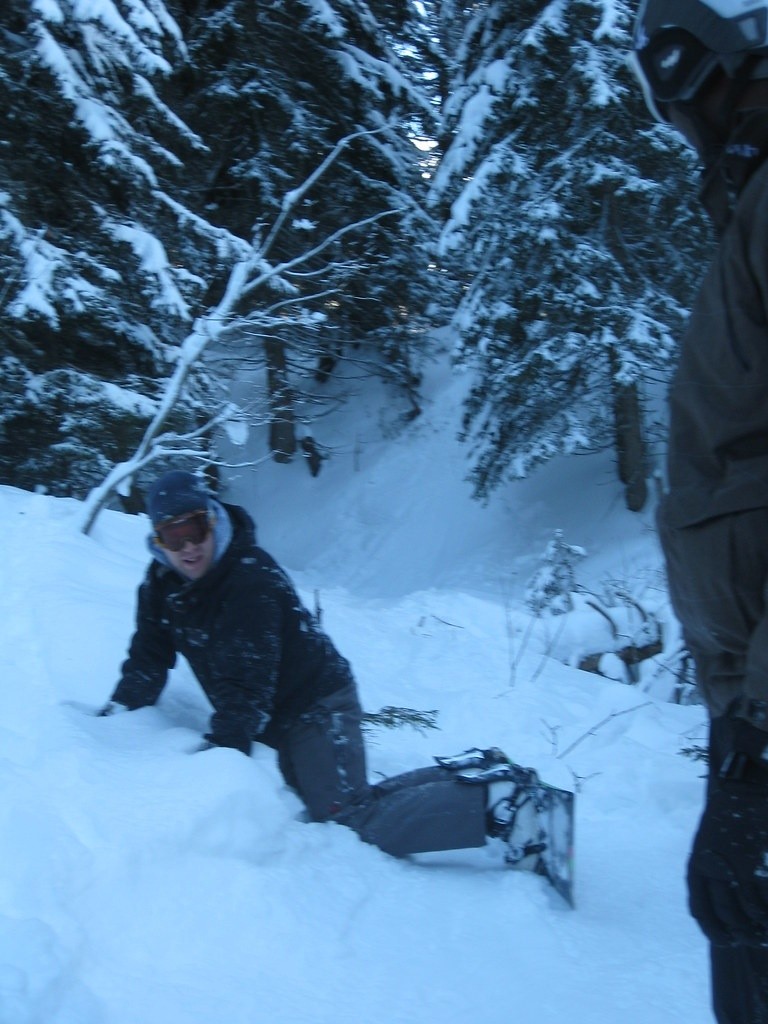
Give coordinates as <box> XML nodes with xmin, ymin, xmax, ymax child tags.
<box><xmin>622</xmin><ymin>0</ymin><xmax>768</xmax><ymax>1024</ymax></box>
<box><xmin>100</xmin><ymin>470</ymin><xmax>578</xmax><ymax>909</ymax></box>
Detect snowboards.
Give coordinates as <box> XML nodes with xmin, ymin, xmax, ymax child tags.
<box><xmin>530</xmin><ymin>785</ymin><xmax>577</xmax><ymax>928</ymax></box>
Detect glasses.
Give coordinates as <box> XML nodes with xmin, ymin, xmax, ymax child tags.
<box><xmin>158</xmin><ymin>516</ymin><xmax>208</xmax><ymax>552</ymax></box>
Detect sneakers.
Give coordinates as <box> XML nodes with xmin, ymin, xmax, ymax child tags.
<box><xmin>433</xmin><ymin>746</ymin><xmax>509</xmax><ymax>768</ymax></box>
<box><xmin>454</xmin><ymin>761</ymin><xmax>550</xmax><ymax>875</ymax></box>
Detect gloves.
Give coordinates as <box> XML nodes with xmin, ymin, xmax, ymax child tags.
<box><xmin>96</xmin><ymin>700</ymin><xmax>132</xmax><ymax>717</ymax></box>
<box><xmin>204</xmin><ymin>701</ymin><xmax>263</xmax><ymax>757</ymax></box>
<box><xmin>684</xmin><ymin>760</ymin><xmax>768</xmax><ymax>953</ymax></box>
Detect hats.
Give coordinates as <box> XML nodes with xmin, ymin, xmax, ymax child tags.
<box><xmin>147</xmin><ymin>469</ymin><xmax>212</xmax><ymax>529</ymax></box>
